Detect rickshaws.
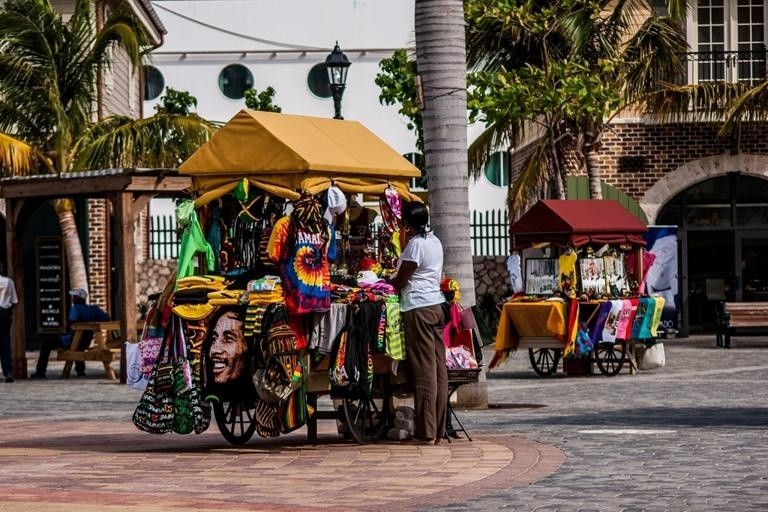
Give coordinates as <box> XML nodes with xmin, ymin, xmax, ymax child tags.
<box><xmin>495</xmin><ymin>199</ymin><xmax>667</xmax><ymax>377</ymax></box>
<box><xmin>149</xmin><ymin>107</ymin><xmax>481</xmax><ymax>446</ymax></box>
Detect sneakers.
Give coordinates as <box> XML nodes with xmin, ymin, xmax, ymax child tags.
<box><xmin>31</xmin><ymin>372</ymin><xmax>46</xmax><ymax>379</ymax></box>
<box><xmin>77</xmin><ymin>371</ymin><xmax>85</xmax><ymax>376</ymax></box>
<box><xmin>401</xmin><ymin>435</ymin><xmax>441</xmax><ymax>445</ymax></box>
<box><xmin>5</xmin><ymin>375</ymin><xmax>14</xmax><ymax>383</ymax></box>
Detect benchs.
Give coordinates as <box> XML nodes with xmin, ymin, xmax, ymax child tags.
<box><xmin>713</xmin><ymin>298</ymin><xmax>767</xmax><ymax>349</ymax></box>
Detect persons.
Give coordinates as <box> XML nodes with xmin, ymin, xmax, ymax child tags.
<box><xmin>380</xmin><ymin>200</ymin><xmax>452</xmax><ymax>446</ymax></box>
<box><xmin>29</xmin><ymin>288</ymin><xmax>114</xmax><ymax>379</ymax></box>
<box><xmin>0</xmin><ymin>275</ymin><xmax>22</xmax><ymax>384</ymax></box>
<box><xmin>197</xmin><ymin>304</ymin><xmax>254</xmax><ymax>402</ymax></box>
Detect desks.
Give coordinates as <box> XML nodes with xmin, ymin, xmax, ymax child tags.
<box><xmin>55</xmin><ymin>319</ymin><xmax>147</xmax><ymax>381</ymax></box>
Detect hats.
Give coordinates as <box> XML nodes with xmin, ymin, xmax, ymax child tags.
<box><xmin>295</xmin><ymin>199</ymin><xmax>322</xmax><ymax>234</ymax></box>
<box><xmin>322</xmin><ymin>187</ymin><xmax>347</xmax><ymax>225</ymax></box>
<box><xmin>69</xmin><ymin>289</ymin><xmax>87</xmax><ymax>299</ymax></box>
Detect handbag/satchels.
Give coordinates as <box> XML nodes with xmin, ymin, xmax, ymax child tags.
<box><xmin>327</xmin><ymin>324</ymin><xmax>361</xmax><ymax>389</ymax></box>
<box><xmin>635</xmin><ymin>342</ymin><xmax>665</xmax><ymax>371</ymax></box>
<box><xmin>253</xmin><ymin>320</ymin><xmax>308</xmax><ymax>437</ymax></box>
<box><xmin>125</xmin><ymin>336</ymin><xmax>212</xmax><ymax>435</ymax></box>
